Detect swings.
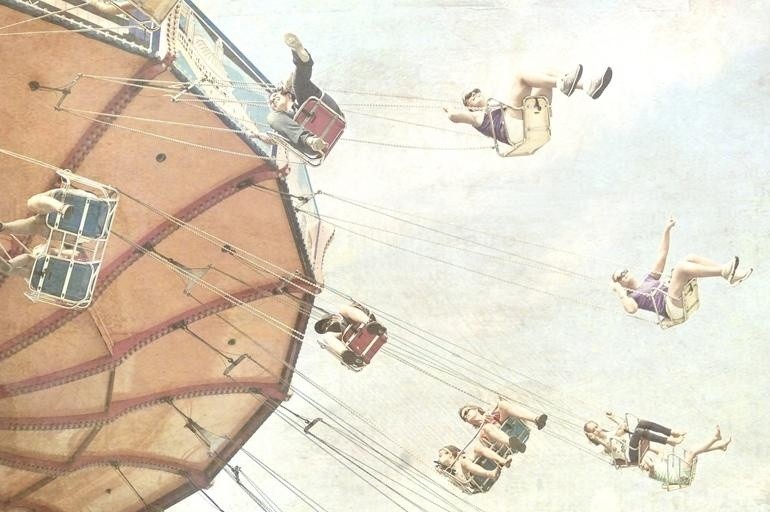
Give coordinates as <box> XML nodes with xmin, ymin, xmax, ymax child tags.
<box><xmin>55</xmin><ymin>72</ymin><xmax>347</xmax><ymax>168</ymax></box>
<box><xmin>279</xmin><ymin>271</ymin><xmax>698</xmax><ymax>493</ymax></box>
<box><xmin>169</xmin><ymin>74</ymin><xmax>555</xmax><ymax>157</ymax></box>
<box><xmin>296</xmin><ymin>188</ymin><xmax>699</xmax><ymax>329</ymax></box>
<box><xmin>183</xmin><ymin>264</ymin><xmax>532</xmax><ymax>495</ymax></box>
<box><xmin>102</xmin><ymin>187</ymin><xmax>390</xmax><ymax>373</ymax></box>
<box><xmin>0</xmin><ymin>150</ymin><xmax>120</xmax><ymax>310</ymax></box>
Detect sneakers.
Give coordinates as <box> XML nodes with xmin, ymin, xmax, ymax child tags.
<box><xmin>307</xmin><ymin>135</ymin><xmax>327</xmax><ymax>151</ymax></box>
<box><xmin>558</xmin><ymin>63</ymin><xmax>583</xmax><ymax>96</ymax></box>
<box><xmin>536</xmin><ymin>414</ymin><xmax>547</xmax><ymax>430</ymax></box>
<box><xmin>588</xmin><ymin>67</ymin><xmax>612</xmax><ymax>99</ymax></box>
<box><xmin>720</xmin><ymin>255</ymin><xmax>752</xmax><ymax>287</ymax></box>
<box><xmin>366</xmin><ymin>321</ymin><xmax>387</xmax><ymax>336</ymax></box>
<box><xmin>341</xmin><ymin>350</ymin><xmax>364</xmax><ymax>367</ymax></box>
<box><xmin>57</xmin><ymin>202</ymin><xmax>76</xmax><ymax>219</ymax></box>
<box><xmin>509</xmin><ymin>436</ymin><xmax>527</xmax><ymax>455</ymax></box>
<box><xmin>284</xmin><ymin>31</ymin><xmax>310</xmax><ymax>63</ymax></box>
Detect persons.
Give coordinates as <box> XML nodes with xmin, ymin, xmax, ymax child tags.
<box><xmin>0</xmin><ymin>235</ymin><xmax>91</xmax><ymax>281</ymax></box>
<box><xmin>609</xmin><ymin>218</ymin><xmax>754</xmax><ymax>316</ymax></box>
<box><xmin>435</xmin><ymin>444</ymin><xmax>514</xmax><ymax>481</ymax></box>
<box><xmin>639</xmin><ymin>424</ymin><xmax>732</xmax><ymax>484</ymax></box>
<box><xmin>314</xmin><ymin>305</ymin><xmax>388</xmax><ymax>365</ymax></box>
<box><xmin>247</xmin><ymin>30</ymin><xmax>345</xmax><ymax>159</ymax></box>
<box><xmin>457</xmin><ymin>398</ymin><xmax>548</xmax><ymax>454</ymax></box>
<box><xmin>583</xmin><ymin>412</ymin><xmax>683</xmax><ymax>467</ymax></box>
<box><xmin>438</xmin><ymin>63</ymin><xmax>613</xmax><ymax>146</ymax></box>
<box><xmin>1</xmin><ymin>188</ymin><xmax>96</xmax><ymax>243</ymax></box>
